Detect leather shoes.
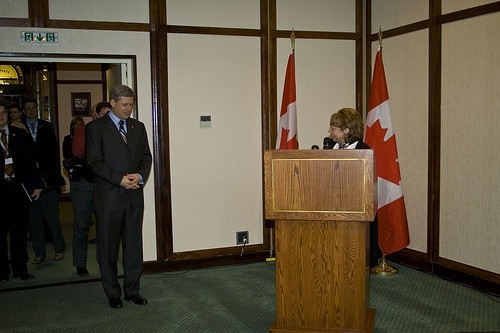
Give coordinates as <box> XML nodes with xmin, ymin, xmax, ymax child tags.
<box><xmin>109</xmin><ymin>297</ymin><xmax>123</xmax><ymax>308</ymax></box>
<box><xmin>124</xmin><ymin>295</ymin><xmax>148</xmax><ymax>305</ymax></box>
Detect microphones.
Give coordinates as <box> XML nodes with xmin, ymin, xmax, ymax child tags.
<box><xmin>312</xmin><ymin>145</ymin><xmax>319</xmax><ymax>150</ymax></box>
<box><xmin>324</xmin><ymin>137</ymin><xmax>331</xmax><ymax>149</ymax></box>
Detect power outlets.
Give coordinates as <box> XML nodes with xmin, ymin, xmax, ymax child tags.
<box><xmin>236</xmin><ymin>231</ymin><xmax>249</xmax><ymax>245</ymax></box>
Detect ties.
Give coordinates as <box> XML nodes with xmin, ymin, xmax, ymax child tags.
<box><xmin>118</xmin><ymin>120</ymin><xmax>128</xmax><ymax>147</ymax></box>
<box><xmin>0</xmin><ymin>129</ymin><xmax>14</xmax><ymax>177</ymax></box>
<box><xmin>29</xmin><ymin>122</ymin><xmax>35</xmax><ymax>135</ymax></box>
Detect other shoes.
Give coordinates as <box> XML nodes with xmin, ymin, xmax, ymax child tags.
<box><xmin>31</xmin><ymin>256</ymin><xmax>45</xmax><ymax>264</ymax></box>
<box><xmin>13</xmin><ymin>271</ymin><xmax>35</xmax><ymax>280</ymax></box>
<box><xmin>77</xmin><ymin>267</ymin><xmax>89</xmax><ymax>276</ymax></box>
<box><xmin>0</xmin><ymin>276</ymin><xmax>9</xmax><ymax>282</ymax></box>
<box><xmin>54</xmin><ymin>253</ymin><xmax>64</xmax><ymax>261</ymax></box>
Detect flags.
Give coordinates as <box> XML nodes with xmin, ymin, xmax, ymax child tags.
<box><xmin>275</xmin><ymin>52</ymin><xmax>300</xmax><ymax>150</ymax></box>
<box><xmin>362</xmin><ymin>50</ymin><xmax>410</xmax><ymax>254</ymax></box>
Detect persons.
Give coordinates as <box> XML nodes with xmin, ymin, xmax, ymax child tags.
<box><xmin>0</xmin><ymin>98</ymin><xmax>112</xmax><ymax>281</ymax></box>
<box><xmin>83</xmin><ymin>85</ymin><xmax>152</xmax><ymax>309</ymax></box>
<box><xmin>323</xmin><ymin>108</ymin><xmax>371</xmax><ymax>150</ymax></box>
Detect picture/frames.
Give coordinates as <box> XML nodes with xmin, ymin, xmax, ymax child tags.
<box><xmin>70</xmin><ymin>90</ymin><xmax>92</xmax><ymax>117</ymax></box>
